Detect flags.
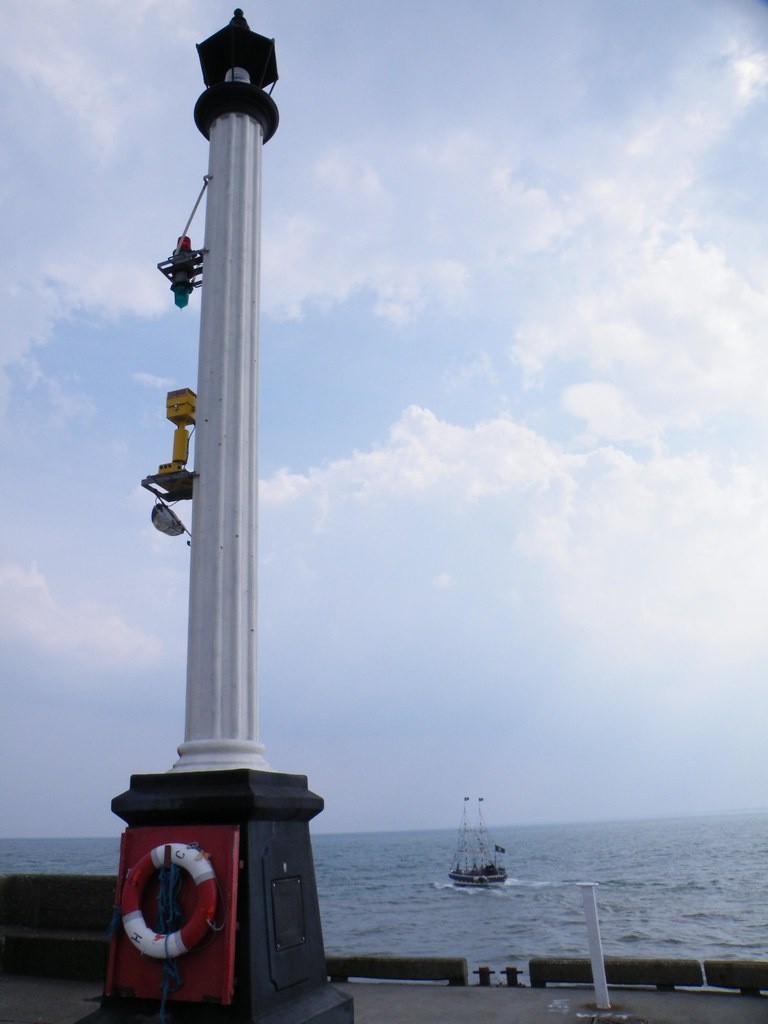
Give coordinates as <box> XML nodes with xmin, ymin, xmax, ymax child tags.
<box><xmin>495</xmin><ymin>845</ymin><xmax>505</xmax><ymax>853</ymax></box>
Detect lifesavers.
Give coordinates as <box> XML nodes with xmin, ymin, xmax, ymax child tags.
<box><xmin>121</xmin><ymin>842</ymin><xmax>218</xmax><ymax>960</ymax></box>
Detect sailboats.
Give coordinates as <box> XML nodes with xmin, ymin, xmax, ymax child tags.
<box><xmin>448</xmin><ymin>797</ymin><xmax>509</xmax><ymax>889</ymax></box>
